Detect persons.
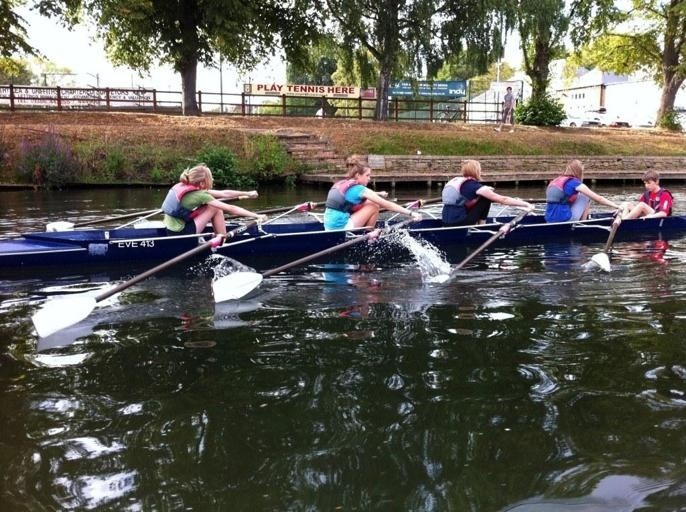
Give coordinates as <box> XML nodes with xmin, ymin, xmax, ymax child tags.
<box><xmin>161</xmin><ymin>163</ymin><xmax>268</xmax><ymax>242</ymax></box>
<box><xmin>621</xmin><ymin>170</ymin><xmax>674</xmax><ymax>220</ymax></box>
<box><xmin>442</xmin><ymin>160</ymin><xmax>534</xmax><ymax>226</ymax></box>
<box><xmin>545</xmin><ymin>159</ymin><xmax>624</xmax><ymax>221</ymax></box>
<box><xmin>493</xmin><ymin>86</ymin><xmax>515</xmax><ymax>133</ymax></box>
<box><xmin>324</xmin><ymin>155</ymin><xmax>422</xmax><ymax>230</ymax></box>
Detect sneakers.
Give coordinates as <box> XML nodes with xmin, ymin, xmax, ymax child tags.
<box><xmin>493</xmin><ymin>128</ymin><xmax>501</xmax><ymax>133</ymax></box>
<box><xmin>508</xmin><ymin>128</ymin><xmax>514</xmax><ymax>133</ymax></box>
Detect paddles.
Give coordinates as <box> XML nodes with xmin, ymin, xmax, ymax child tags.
<box><xmin>212</xmin><ymin>218</ymin><xmax>421</xmax><ymax>303</ymax></box>
<box><xmin>591</xmin><ymin>212</ymin><xmax>625</xmax><ymax>272</ymax></box>
<box><xmin>428</xmin><ymin>207</ymin><xmax>535</xmax><ymax>283</ymax></box>
<box><xmin>46</xmin><ymin>194</ymin><xmax>250</xmax><ymax>233</ymax></box>
<box><xmin>31</xmin><ymin>218</ymin><xmax>266</xmax><ymax>337</ymax></box>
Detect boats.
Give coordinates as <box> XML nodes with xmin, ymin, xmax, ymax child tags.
<box><xmin>0</xmin><ymin>212</ymin><xmax>685</xmax><ymax>277</ymax></box>
